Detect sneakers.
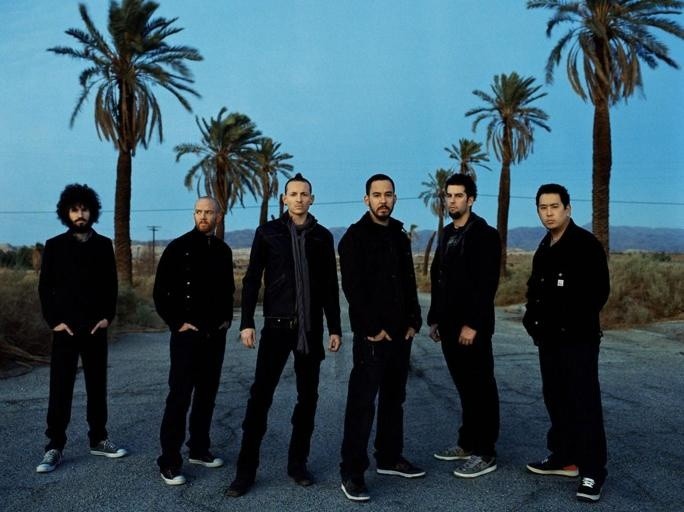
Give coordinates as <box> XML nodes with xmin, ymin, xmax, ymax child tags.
<box><xmin>526</xmin><ymin>452</ymin><xmax>579</xmax><ymax>477</ymax></box>
<box><xmin>288</xmin><ymin>466</ymin><xmax>313</xmax><ymax>486</ymax></box>
<box><xmin>376</xmin><ymin>459</ymin><xmax>426</xmax><ymax>478</ymax></box>
<box><xmin>453</xmin><ymin>454</ymin><xmax>498</xmax><ymax>478</ymax></box>
<box><xmin>433</xmin><ymin>443</ymin><xmax>474</xmax><ymax>461</ymax></box>
<box><xmin>160</xmin><ymin>467</ymin><xmax>187</xmax><ymax>485</ymax></box>
<box><xmin>340</xmin><ymin>473</ymin><xmax>371</xmax><ymax>501</ymax></box>
<box><xmin>36</xmin><ymin>449</ymin><xmax>63</xmax><ymax>473</ymax></box>
<box><xmin>188</xmin><ymin>454</ymin><xmax>224</xmax><ymax>468</ymax></box>
<box><xmin>90</xmin><ymin>438</ymin><xmax>128</xmax><ymax>458</ymax></box>
<box><xmin>227</xmin><ymin>475</ymin><xmax>255</xmax><ymax>496</ymax></box>
<box><xmin>575</xmin><ymin>468</ymin><xmax>609</xmax><ymax>502</ymax></box>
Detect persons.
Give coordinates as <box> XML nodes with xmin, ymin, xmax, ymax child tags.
<box><xmin>523</xmin><ymin>183</ymin><xmax>610</xmax><ymax>503</ymax></box>
<box><xmin>36</xmin><ymin>183</ymin><xmax>129</xmax><ymax>474</ymax></box>
<box><xmin>152</xmin><ymin>197</ymin><xmax>236</xmax><ymax>486</ymax></box>
<box><xmin>225</xmin><ymin>176</ymin><xmax>342</xmax><ymax>500</ymax></box>
<box><xmin>427</xmin><ymin>174</ymin><xmax>502</xmax><ymax>479</ymax></box>
<box><xmin>338</xmin><ymin>173</ymin><xmax>426</xmax><ymax>501</ymax></box>
